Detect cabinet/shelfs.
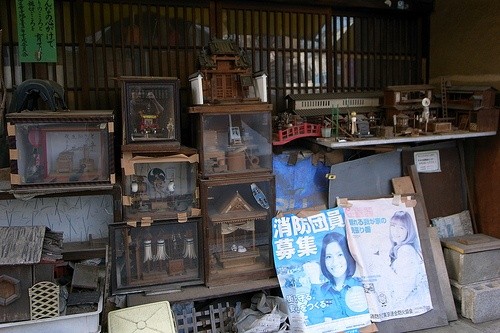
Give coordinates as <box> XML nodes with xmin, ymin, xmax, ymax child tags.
<box><xmin>0</xmin><ymin>103</ymin><xmax>500</xmax><ymax>307</ymax></box>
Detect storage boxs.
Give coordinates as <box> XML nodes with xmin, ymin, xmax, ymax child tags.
<box><xmin>0</xmin><ymin>295</ymin><xmax>104</xmax><ymax>332</ymax></box>
<box><xmin>439</xmin><ymin>233</ymin><xmax>500</xmax><ymax>324</ymax></box>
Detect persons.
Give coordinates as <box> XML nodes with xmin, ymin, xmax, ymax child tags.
<box><xmin>306</xmin><ymin>232</ymin><xmax>369</xmax><ymax>322</ymax></box>
<box><xmin>388</xmin><ymin>211</ymin><xmax>427</xmax><ymax>308</ymax></box>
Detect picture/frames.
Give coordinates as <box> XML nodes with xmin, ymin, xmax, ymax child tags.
<box><xmin>121</xmin><ymin>76</ymin><xmax>181</xmax><ymax>152</ymax></box>
